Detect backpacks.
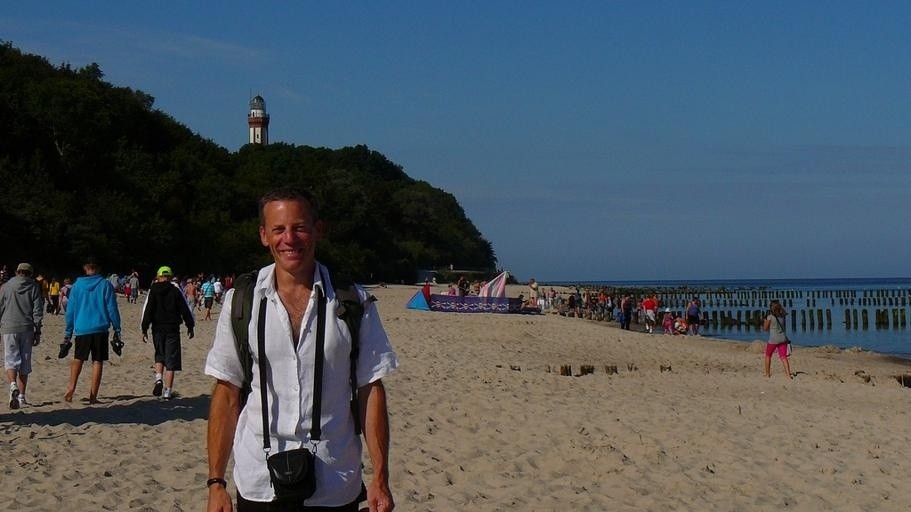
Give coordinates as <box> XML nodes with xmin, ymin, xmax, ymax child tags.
<box><xmin>63</xmin><ymin>286</ymin><xmax>72</xmax><ymax>299</ymax></box>
<box><xmin>34</xmin><ymin>278</ymin><xmax>45</xmax><ymax>293</ymax></box>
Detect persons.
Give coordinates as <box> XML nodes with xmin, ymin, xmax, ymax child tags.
<box><xmin>0</xmin><ymin>255</ymin><xmax>235</xmax><ymax>410</ymax></box>
<box><xmin>423</xmin><ymin>276</ymin><xmax>707</xmax><ymax>337</ymax></box>
<box><xmin>764</xmin><ymin>299</ymin><xmax>793</xmax><ymax>380</ymax></box>
<box><xmin>204</xmin><ymin>185</ymin><xmax>398</xmax><ymax>511</ymax></box>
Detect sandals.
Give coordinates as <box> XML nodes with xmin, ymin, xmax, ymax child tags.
<box><xmin>163</xmin><ymin>397</ymin><xmax>172</xmax><ymax>402</ymax></box>
<box><xmin>153</xmin><ymin>379</ymin><xmax>163</xmax><ymax>396</ymax></box>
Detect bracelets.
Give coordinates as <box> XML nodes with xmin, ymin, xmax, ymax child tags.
<box><xmin>206</xmin><ymin>478</ymin><xmax>227</xmax><ymax>488</ymax></box>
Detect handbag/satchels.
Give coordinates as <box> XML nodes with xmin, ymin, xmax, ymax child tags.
<box><xmin>47</xmin><ymin>304</ymin><xmax>53</xmax><ymax>313</ymax></box>
<box><xmin>199</xmin><ymin>296</ymin><xmax>205</xmax><ymax>306</ymax></box>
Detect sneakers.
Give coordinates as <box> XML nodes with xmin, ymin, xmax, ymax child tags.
<box><xmin>58</xmin><ymin>342</ymin><xmax>72</xmax><ymax>358</ymax></box>
<box><xmin>18</xmin><ymin>397</ymin><xmax>26</xmax><ymax>406</ymax></box>
<box><xmin>110</xmin><ymin>339</ymin><xmax>124</xmax><ymax>356</ymax></box>
<box><xmin>9</xmin><ymin>386</ymin><xmax>20</xmax><ymax>409</ymax></box>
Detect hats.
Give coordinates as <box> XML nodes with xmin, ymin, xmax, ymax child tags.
<box><xmin>64</xmin><ymin>279</ymin><xmax>71</xmax><ymax>284</ymax></box>
<box><xmin>17</xmin><ymin>263</ymin><xmax>34</xmax><ymax>275</ymax></box>
<box><xmin>187</xmin><ymin>279</ymin><xmax>193</xmax><ymax>283</ymax></box>
<box><xmin>172</xmin><ymin>277</ymin><xmax>178</xmax><ymax>282</ymax></box>
<box><xmin>157</xmin><ymin>266</ymin><xmax>173</xmax><ymax>280</ymax></box>
<box><xmin>131</xmin><ymin>272</ymin><xmax>138</xmax><ymax>277</ymax></box>
